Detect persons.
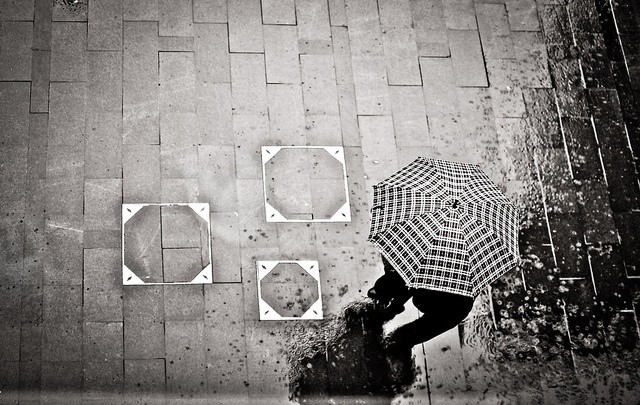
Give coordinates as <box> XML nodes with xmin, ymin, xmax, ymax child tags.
<box><xmin>368</xmin><ymin>261</ymin><xmax>415</xmax><ymax>318</ymax></box>
<box><xmin>391</xmin><ymin>288</ymin><xmax>475</xmax><ymax>369</ymax></box>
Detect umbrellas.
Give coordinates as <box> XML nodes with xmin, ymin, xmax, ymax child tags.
<box><xmin>365</xmin><ymin>154</ymin><xmax>523</xmax><ymax>301</ymax></box>
